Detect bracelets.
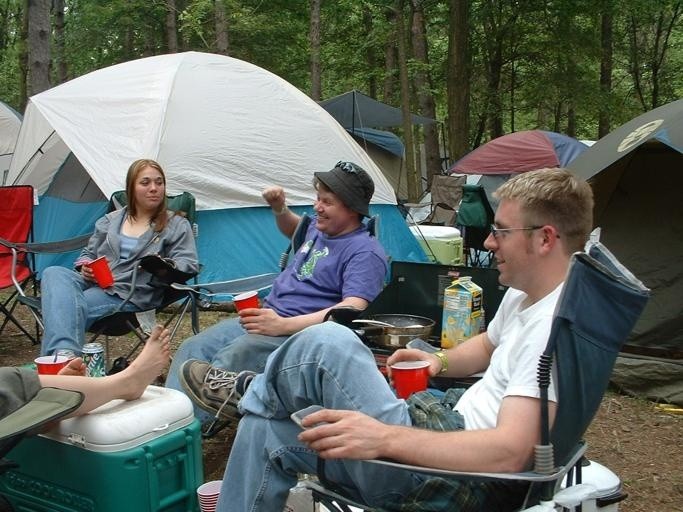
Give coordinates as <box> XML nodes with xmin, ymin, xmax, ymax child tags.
<box><xmin>433</xmin><ymin>351</ymin><xmax>449</xmax><ymax>377</ymax></box>
<box><xmin>270</xmin><ymin>205</ymin><xmax>290</xmax><ymax>217</ymax></box>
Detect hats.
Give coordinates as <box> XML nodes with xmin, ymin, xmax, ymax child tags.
<box><xmin>314</xmin><ymin>161</ymin><xmax>374</xmax><ymax>218</ymax></box>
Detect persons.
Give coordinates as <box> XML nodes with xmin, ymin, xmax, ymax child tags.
<box><xmin>178</xmin><ymin>164</ymin><xmax>598</xmax><ymax>512</ymax></box>
<box><xmin>162</xmin><ymin>160</ymin><xmax>388</xmax><ymax>431</ymax></box>
<box><xmin>1</xmin><ymin>320</ymin><xmax>171</xmax><ymax>464</ymax></box>
<box><xmin>36</xmin><ymin>158</ymin><xmax>201</xmax><ymax>358</ymax></box>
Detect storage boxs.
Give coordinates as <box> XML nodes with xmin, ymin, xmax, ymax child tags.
<box><xmin>1</xmin><ymin>390</ymin><xmax>204</xmax><ymax>509</ymax></box>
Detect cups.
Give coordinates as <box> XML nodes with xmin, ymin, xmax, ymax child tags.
<box><xmin>85</xmin><ymin>254</ymin><xmax>113</xmax><ymax>290</ymax></box>
<box><xmin>231</xmin><ymin>290</ymin><xmax>259</xmax><ymax>318</ymax></box>
<box><xmin>32</xmin><ymin>355</ymin><xmax>68</xmax><ymax>375</ymax></box>
<box><xmin>388</xmin><ymin>361</ymin><xmax>430</xmax><ymax>399</ymax></box>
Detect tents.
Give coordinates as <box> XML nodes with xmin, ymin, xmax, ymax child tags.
<box><xmin>345</xmin><ymin>126</ymin><xmax>410</xmax><ymax>214</ymax></box>
<box><xmin>2</xmin><ymin>48</ymin><xmax>442</xmax><ymax>306</ymax></box>
<box><xmin>0</xmin><ymin>99</ymin><xmax>24</xmax><ymax>188</ymax></box>
<box><xmin>562</xmin><ymin>96</ymin><xmax>683</xmax><ymax>411</ymax></box>
<box><xmin>403</xmin><ymin>129</ymin><xmax>593</xmax><ymax>270</ymax></box>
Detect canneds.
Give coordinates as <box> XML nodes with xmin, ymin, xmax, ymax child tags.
<box><xmin>81</xmin><ymin>343</ymin><xmax>106</xmax><ymax>377</ymax></box>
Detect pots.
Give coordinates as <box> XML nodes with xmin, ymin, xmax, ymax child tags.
<box><xmin>352</xmin><ymin>312</ymin><xmax>435</xmax><ymax>348</ymax></box>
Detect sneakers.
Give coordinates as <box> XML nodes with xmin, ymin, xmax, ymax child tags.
<box><xmin>179</xmin><ymin>358</ymin><xmax>257</xmax><ymax>421</ymax></box>
<box><xmin>51</xmin><ymin>349</ymin><xmax>77</xmax><ymax>362</ymax></box>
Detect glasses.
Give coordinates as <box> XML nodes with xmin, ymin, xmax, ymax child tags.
<box><xmin>491</xmin><ymin>225</ymin><xmax>560</xmax><ymax>239</ymax></box>
<box><xmin>335</xmin><ymin>161</ymin><xmax>366</xmax><ymax>184</ymax></box>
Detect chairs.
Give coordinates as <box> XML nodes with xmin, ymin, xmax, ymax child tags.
<box><xmin>0</xmin><ymin>184</ymin><xmax>198</xmax><ymax>371</ymax></box>
<box><xmin>403</xmin><ymin>176</ymin><xmax>495</xmax><ymax>267</ymax></box>
<box><xmin>309</xmin><ymin>240</ymin><xmax>652</xmax><ymax>511</ymax></box>
